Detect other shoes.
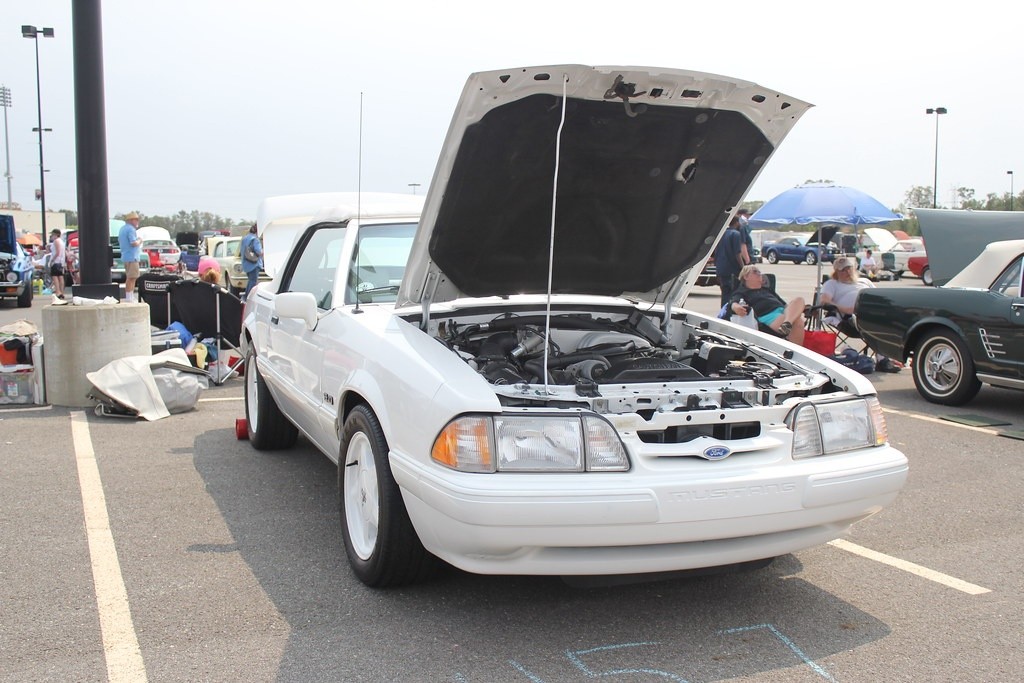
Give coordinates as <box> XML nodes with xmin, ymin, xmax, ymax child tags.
<box><xmin>874</xmin><ymin>359</ymin><xmax>902</xmax><ymax>373</ymax></box>
<box><xmin>57</xmin><ymin>292</ymin><xmax>64</xmax><ymax>300</ymax></box>
<box><xmin>778</xmin><ymin>322</ymin><xmax>792</xmax><ymax>336</ymax></box>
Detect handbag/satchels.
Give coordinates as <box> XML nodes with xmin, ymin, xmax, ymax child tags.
<box><xmin>803</xmin><ymin>315</ymin><xmax>837</xmax><ymax>358</ymax></box>
<box><xmin>244</xmin><ymin>238</ymin><xmax>259</xmax><ymax>263</ymax></box>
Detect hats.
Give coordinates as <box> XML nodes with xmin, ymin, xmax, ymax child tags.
<box><xmin>867</xmin><ymin>250</ymin><xmax>872</xmax><ymax>255</ymax></box>
<box><xmin>833</xmin><ymin>255</ymin><xmax>854</xmax><ymax>272</ymax></box>
<box><xmin>737</xmin><ymin>208</ymin><xmax>754</xmax><ymax>216</ymax></box>
<box><xmin>124</xmin><ymin>212</ymin><xmax>139</xmax><ymax>221</ymax></box>
<box><xmin>48</xmin><ymin>229</ymin><xmax>61</xmax><ymax>237</ymax></box>
<box><xmin>198</xmin><ymin>255</ymin><xmax>221</xmax><ymax>276</ymax></box>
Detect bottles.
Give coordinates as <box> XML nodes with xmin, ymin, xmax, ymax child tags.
<box><xmin>739</xmin><ymin>297</ymin><xmax>746</xmax><ymax>306</ymax></box>
<box><xmin>38</xmin><ymin>277</ymin><xmax>44</xmax><ymax>297</ymax></box>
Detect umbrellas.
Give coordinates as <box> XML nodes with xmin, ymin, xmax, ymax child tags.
<box><xmin>749</xmin><ymin>179</ymin><xmax>903</xmax><ymax>305</ymax></box>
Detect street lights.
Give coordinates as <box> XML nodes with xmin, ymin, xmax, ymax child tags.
<box><xmin>927</xmin><ymin>107</ymin><xmax>948</xmax><ymax>209</ymax></box>
<box><xmin>21</xmin><ymin>24</ymin><xmax>55</xmax><ymax>248</ymax></box>
<box><xmin>1006</xmin><ymin>170</ymin><xmax>1013</xmax><ymax>211</ymax></box>
<box><xmin>0</xmin><ymin>86</ymin><xmax>14</xmax><ymax>211</ymax></box>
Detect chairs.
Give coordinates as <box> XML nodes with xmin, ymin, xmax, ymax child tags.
<box><xmin>32</xmin><ymin>254</ymin><xmax>53</xmax><ymax>289</ymax></box>
<box><xmin>137</xmin><ymin>268</ymin><xmax>252</xmax><ymax>387</ymax></box>
<box><xmin>721</xmin><ymin>253</ymin><xmax>904</xmax><ymax>372</ymax></box>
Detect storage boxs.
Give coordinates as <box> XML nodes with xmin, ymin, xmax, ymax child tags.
<box><xmin>150</xmin><ymin>330</ymin><xmax>183</xmax><ymax>357</ymax></box>
<box><xmin>0</xmin><ymin>334</ymin><xmax>45</xmax><ymax>406</ymax></box>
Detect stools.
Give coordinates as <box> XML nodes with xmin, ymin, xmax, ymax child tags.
<box><xmin>34</xmin><ymin>279</ymin><xmax>44</xmax><ymax>296</ymax></box>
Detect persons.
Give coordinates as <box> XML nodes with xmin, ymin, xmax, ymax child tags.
<box><xmin>821</xmin><ymin>256</ymin><xmax>901</xmax><ymax>373</ymax></box>
<box><xmin>197</xmin><ymin>257</ymin><xmax>221</xmax><ymax>283</ymax></box>
<box><xmin>241</xmin><ymin>224</ymin><xmax>264</xmax><ymax>300</ymax></box>
<box><xmin>118</xmin><ymin>211</ymin><xmax>142</xmax><ymax>299</ymax></box>
<box><xmin>711</xmin><ymin>209</ymin><xmax>757</xmax><ymax>310</ymax></box>
<box><xmin>33</xmin><ymin>229</ymin><xmax>65</xmax><ymax>298</ymax></box>
<box><xmin>860</xmin><ymin>249</ymin><xmax>880</xmax><ymax>282</ymax></box>
<box><xmin>730</xmin><ymin>265</ymin><xmax>805</xmax><ymax>345</ymax></box>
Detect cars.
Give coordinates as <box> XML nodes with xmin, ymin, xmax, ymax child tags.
<box><xmin>893</xmin><ymin>229</ymin><xmax>923</xmax><ymax>250</ymax></box>
<box><xmin>827</xmin><ymin>230</ymin><xmax>874</xmax><ymax>258</ymax></box>
<box><xmin>853</xmin><ymin>206</ymin><xmax>1024</xmax><ymax>407</ymax></box>
<box><xmin>908</xmin><ymin>254</ymin><xmax>934</xmax><ymax>287</ymax></box>
<box><xmin>239</xmin><ymin>65</ymin><xmax>911</xmax><ymax>592</ymax></box>
<box><xmin>856</xmin><ymin>226</ymin><xmax>927</xmax><ymax>276</ymax></box>
<box><xmin>0</xmin><ymin>212</ymin><xmax>35</xmax><ymax>310</ymax></box>
<box><xmin>760</xmin><ymin>225</ymin><xmax>841</xmax><ymax>266</ymax></box>
<box><xmin>106</xmin><ymin>217</ymin><xmax>273</xmax><ymax>299</ymax></box>
<box><xmin>694</xmin><ymin>244</ymin><xmax>763</xmax><ymax>287</ymax></box>
